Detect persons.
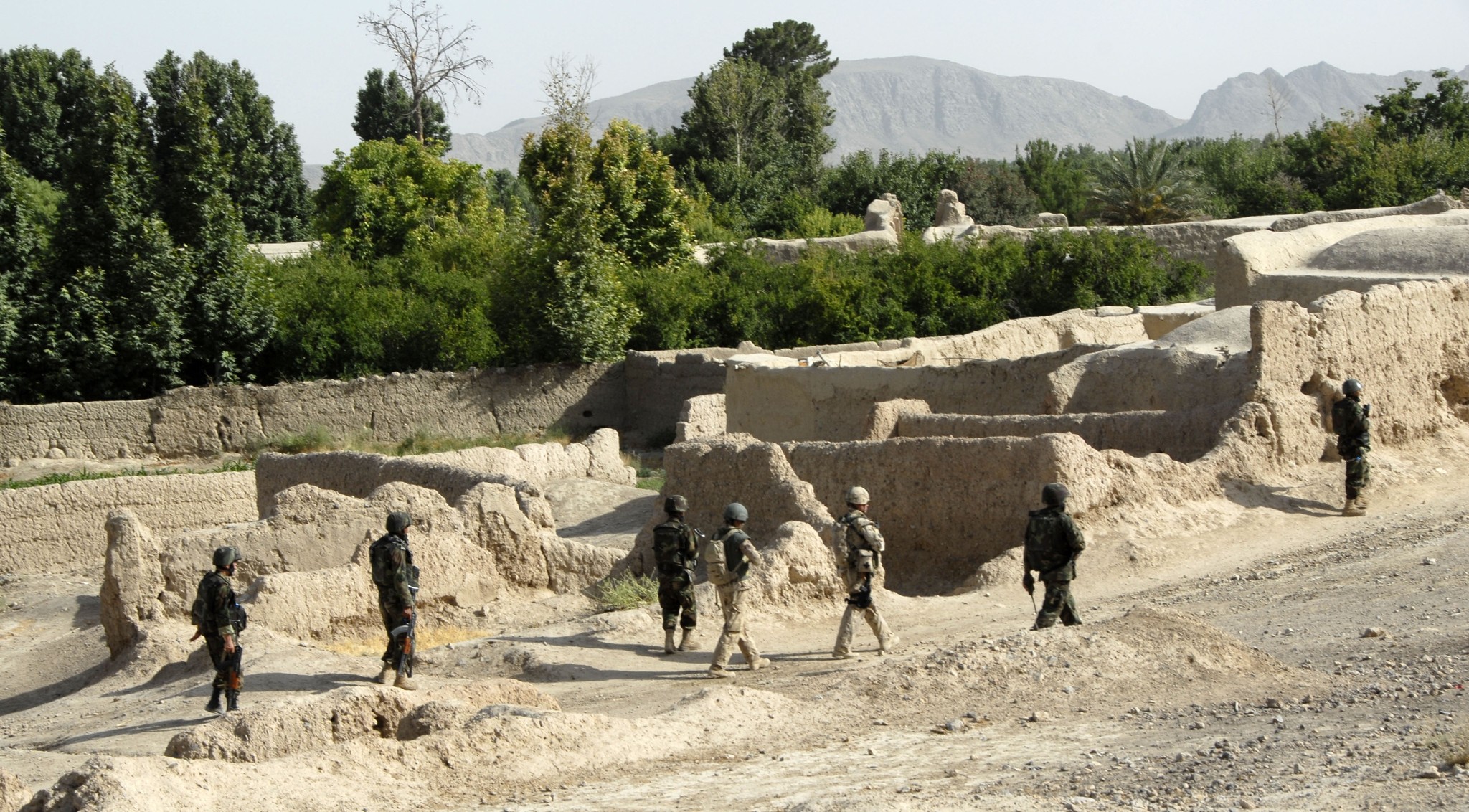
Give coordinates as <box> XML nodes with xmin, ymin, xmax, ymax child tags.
<box><xmin>651</xmin><ymin>495</ymin><xmax>703</xmax><ymax>653</ymax></box>
<box><xmin>369</xmin><ymin>512</ymin><xmax>420</xmax><ymax>690</ymax></box>
<box><xmin>1023</xmin><ymin>484</ymin><xmax>1085</xmax><ymax>631</ymax></box>
<box><xmin>832</xmin><ymin>486</ymin><xmax>900</xmax><ymax>659</ymax></box>
<box><xmin>191</xmin><ymin>547</ymin><xmax>247</xmax><ymax>715</ymax></box>
<box><xmin>709</xmin><ymin>503</ymin><xmax>771</xmax><ymax>677</ymax></box>
<box><xmin>1331</xmin><ymin>379</ymin><xmax>1370</xmax><ymax>517</ymax></box>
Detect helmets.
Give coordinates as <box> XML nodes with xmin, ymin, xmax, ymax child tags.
<box><xmin>845</xmin><ymin>486</ymin><xmax>870</xmax><ymax>505</ymax></box>
<box><xmin>724</xmin><ymin>502</ymin><xmax>749</xmax><ymax>522</ymax></box>
<box><xmin>1343</xmin><ymin>380</ymin><xmax>1362</xmax><ymax>393</ymax></box>
<box><xmin>213</xmin><ymin>547</ymin><xmax>242</xmax><ymax>566</ymax></box>
<box><xmin>1042</xmin><ymin>483</ymin><xmax>1071</xmax><ymax>504</ymax></box>
<box><xmin>386</xmin><ymin>512</ymin><xmax>413</xmax><ymax>530</ymax></box>
<box><xmin>663</xmin><ymin>495</ymin><xmax>689</xmax><ymax>513</ymax></box>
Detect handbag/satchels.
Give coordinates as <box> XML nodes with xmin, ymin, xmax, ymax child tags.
<box><xmin>858</xmin><ymin>549</ymin><xmax>874</xmax><ymax>573</ymax></box>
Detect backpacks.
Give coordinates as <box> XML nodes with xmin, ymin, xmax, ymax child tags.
<box><xmin>1028</xmin><ymin>512</ymin><xmax>1069</xmax><ymax>572</ymax></box>
<box><xmin>705</xmin><ymin>529</ymin><xmax>749</xmax><ymax>584</ymax></box>
<box><xmin>832</xmin><ymin>516</ymin><xmax>872</xmax><ymax>568</ymax></box>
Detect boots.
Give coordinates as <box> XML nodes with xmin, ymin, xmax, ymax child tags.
<box><xmin>665</xmin><ymin>629</ymin><xmax>677</xmax><ymax>653</ymax></box>
<box><xmin>1341</xmin><ymin>496</ymin><xmax>1368</xmax><ymax>516</ymax></box>
<box><xmin>204</xmin><ymin>688</ymin><xmax>224</xmax><ymax>715</ymax></box>
<box><xmin>225</xmin><ymin>691</ymin><xmax>240</xmax><ymax>711</ymax></box>
<box><xmin>749</xmin><ymin>658</ymin><xmax>771</xmax><ymax>670</ymax></box>
<box><xmin>393</xmin><ymin>671</ymin><xmax>416</xmax><ymax>690</ymax></box>
<box><xmin>707</xmin><ymin>664</ymin><xmax>736</xmax><ymax>678</ymax></box>
<box><xmin>677</xmin><ymin>630</ymin><xmax>701</xmax><ymax>651</ymax></box>
<box><xmin>378</xmin><ymin>662</ymin><xmax>391</xmax><ymax>684</ymax></box>
<box><xmin>832</xmin><ymin>647</ymin><xmax>861</xmax><ymax>660</ymax></box>
<box><xmin>880</xmin><ymin>636</ymin><xmax>902</xmax><ymax>652</ymax></box>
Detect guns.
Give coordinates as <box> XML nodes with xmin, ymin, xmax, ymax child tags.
<box><xmin>390</xmin><ymin>609</ymin><xmax>417</xmax><ymax>674</ymax></box>
<box><xmin>224</xmin><ymin>620</ymin><xmax>243</xmax><ymax>710</ymax></box>
<box><xmin>1362</xmin><ymin>401</ymin><xmax>1371</xmax><ymax>418</ymax></box>
<box><xmin>692</xmin><ymin>529</ymin><xmax>705</xmax><ymax>566</ymax></box>
